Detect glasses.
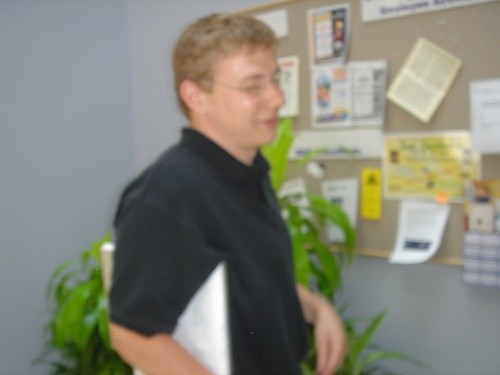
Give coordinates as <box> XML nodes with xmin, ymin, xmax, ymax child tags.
<box><xmin>206</xmin><ymin>69</ymin><xmax>292</xmax><ymax>95</ymax></box>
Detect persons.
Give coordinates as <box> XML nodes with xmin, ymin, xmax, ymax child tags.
<box><xmin>107</xmin><ymin>10</ymin><xmax>350</xmax><ymax>374</ymax></box>
